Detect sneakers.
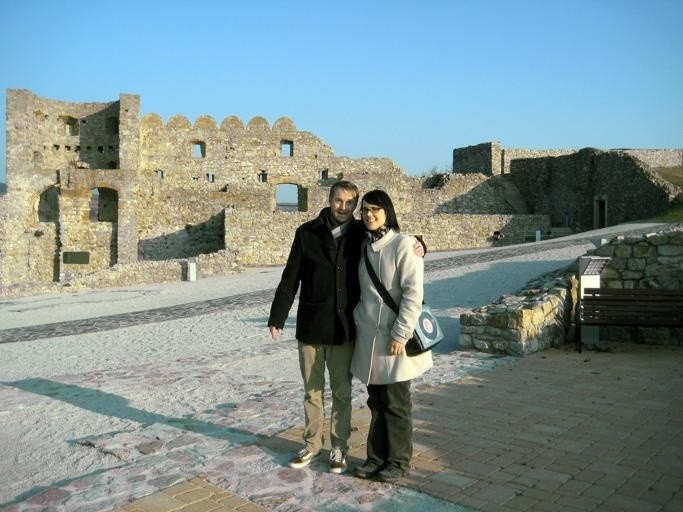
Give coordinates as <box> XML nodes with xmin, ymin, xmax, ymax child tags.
<box><xmin>287</xmin><ymin>448</ymin><xmax>322</xmax><ymax>469</ymax></box>
<box><xmin>328</xmin><ymin>449</ymin><xmax>349</xmax><ymax>474</ymax></box>
<box><xmin>355</xmin><ymin>461</ymin><xmax>380</xmax><ymax>480</ymax></box>
<box><xmin>378</xmin><ymin>464</ymin><xmax>405</xmax><ymax>483</ymax></box>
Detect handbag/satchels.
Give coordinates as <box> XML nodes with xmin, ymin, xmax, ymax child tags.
<box><xmin>405</xmin><ymin>306</ymin><xmax>445</xmax><ymax>357</ymax></box>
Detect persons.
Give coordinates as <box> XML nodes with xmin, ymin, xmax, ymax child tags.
<box><xmin>267</xmin><ymin>180</ymin><xmax>427</xmax><ymax>474</ymax></box>
<box><xmin>346</xmin><ymin>189</ymin><xmax>434</xmax><ymax>481</ymax></box>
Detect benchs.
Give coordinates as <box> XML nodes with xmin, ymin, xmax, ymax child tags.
<box><xmin>572</xmin><ymin>288</ymin><xmax>683</xmax><ymax>353</ymax></box>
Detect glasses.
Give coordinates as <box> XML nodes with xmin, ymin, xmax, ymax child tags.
<box><xmin>359</xmin><ymin>206</ymin><xmax>382</xmax><ymax>215</ymax></box>
